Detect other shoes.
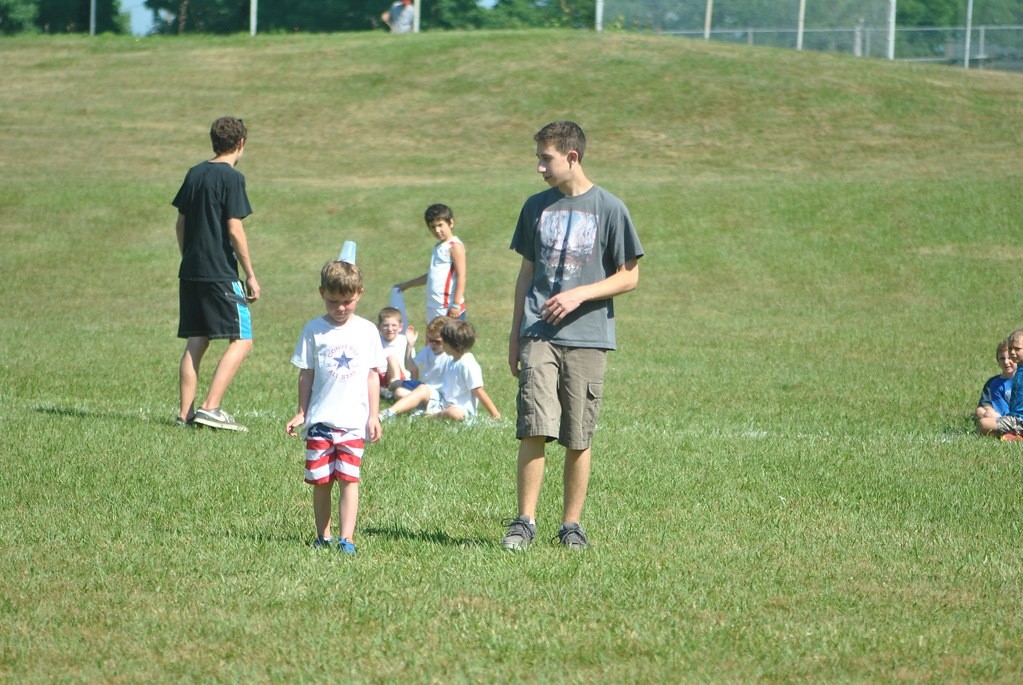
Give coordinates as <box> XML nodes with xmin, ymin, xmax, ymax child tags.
<box><xmin>312</xmin><ymin>535</ymin><xmax>336</xmax><ymax>548</ymax></box>
<box><xmin>1001</xmin><ymin>433</ymin><xmax>1023</xmax><ymax>441</ymax></box>
<box><xmin>338</xmin><ymin>536</ymin><xmax>356</xmax><ymax>553</ymax></box>
<box><xmin>379</xmin><ymin>409</ymin><xmax>394</xmax><ymax>421</ymax></box>
<box><xmin>380</xmin><ymin>388</ymin><xmax>392</xmax><ymax>399</ymax></box>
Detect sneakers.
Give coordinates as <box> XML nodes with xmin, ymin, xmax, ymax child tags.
<box><xmin>501</xmin><ymin>514</ymin><xmax>536</xmax><ymax>550</ymax></box>
<box><xmin>193</xmin><ymin>405</ymin><xmax>247</xmax><ymax>430</ymax></box>
<box><xmin>551</xmin><ymin>522</ymin><xmax>591</xmax><ymax>549</ymax></box>
<box><xmin>175</xmin><ymin>413</ymin><xmax>198</xmax><ymax>427</ymax></box>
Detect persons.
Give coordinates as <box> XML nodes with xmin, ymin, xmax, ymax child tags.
<box><xmin>283</xmin><ymin>260</ymin><xmax>389</xmax><ymax>556</ymax></box>
<box><xmin>378</xmin><ymin>319</ymin><xmax>500</xmax><ymax>423</ymax></box>
<box><xmin>378</xmin><ymin>307</ymin><xmax>416</xmax><ymax>400</ymax></box>
<box><xmin>500</xmin><ymin>120</ymin><xmax>645</xmax><ymax>550</ymax></box>
<box><xmin>389</xmin><ymin>315</ymin><xmax>452</xmax><ymax>415</ymax></box>
<box><xmin>392</xmin><ymin>204</ymin><xmax>468</xmax><ymax>344</ymax></box>
<box><xmin>170</xmin><ymin>117</ymin><xmax>261</xmax><ymax>431</ymax></box>
<box><xmin>381</xmin><ymin>0</ymin><xmax>414</xmax><ymax>34</ymax></box>
<box><xmin>979</xmin><ymin>328</ymin><xmax>1023</xmax><ymax>442</ymax></box>
<box><xmin>975</xmin><ymin>339</ymin><xmax>1018</xmax><ymax>417</ymax></box>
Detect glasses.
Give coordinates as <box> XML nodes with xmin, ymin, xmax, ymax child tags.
<box><xmin>427</xmin><ymin>336</ymin><xmax>443</xmax><ymax>344</ymax></box>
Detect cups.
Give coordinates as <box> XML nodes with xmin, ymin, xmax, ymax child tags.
<box><xmin>338</xmin><ymin>240</ymin><xmax>356</xmax><ymax>266</ymax></box>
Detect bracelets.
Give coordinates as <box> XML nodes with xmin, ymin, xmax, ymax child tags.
<box><xmin>451</xmin><ymin>304</ymin><xmax>460</xmax><ymax>310</ymax></box>
